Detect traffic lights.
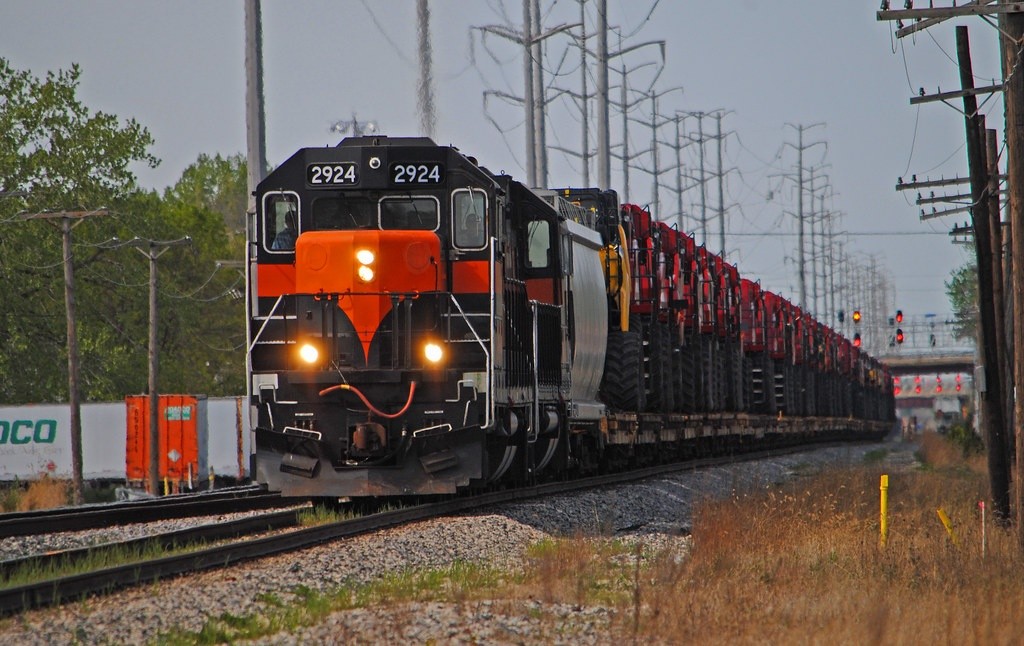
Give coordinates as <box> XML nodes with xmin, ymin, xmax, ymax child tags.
<box><xmin>852</xmin><ymin>333</ymin><xmax>861</xmax><ymax>346</ymax></box>
<box><xmin>896</xmin><ymin>328</ymin><xmax>903</xmax><ymax>344</ymax></box>
<box><xmin>853</xmin><ymin>311</ymin><xmax>860</xmax><ymax>323</ymax></box>
<box><xmin>895</xmin><ymin>310</ymin><xmax>903</xmax><ymax>322</ymax></box>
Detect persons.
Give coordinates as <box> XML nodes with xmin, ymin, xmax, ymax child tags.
<box><xmin>272</xmin><ymin>210</ymin><xmax>299</xmax><ymax>251</ymax></box>
<box><xmin>459</xmin><ymin>213</ymin><xmax>482</xmax><ymax>246</ymax></box>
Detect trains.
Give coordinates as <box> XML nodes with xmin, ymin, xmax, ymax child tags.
<box><xmin>249</xmin><ymin>135</ymin><xmax>897</xmax><ymax>502</ymax></box>
